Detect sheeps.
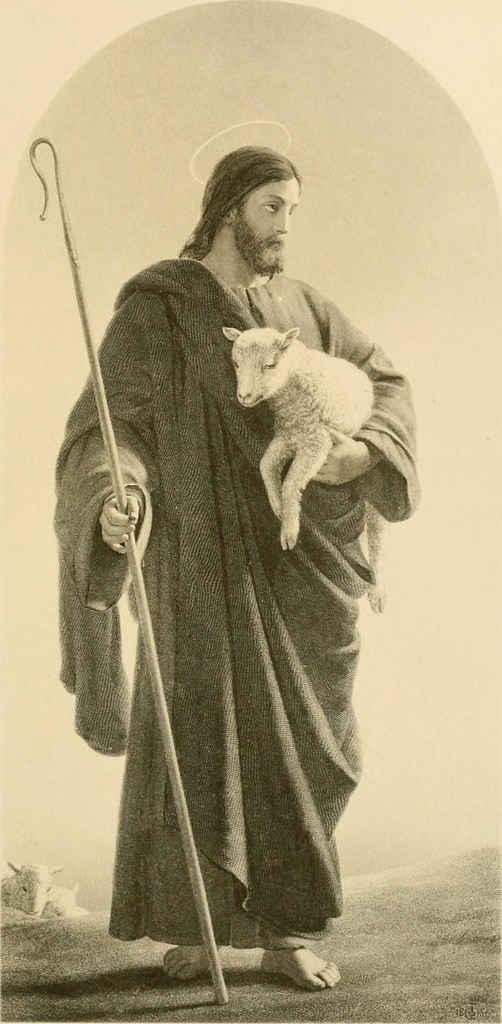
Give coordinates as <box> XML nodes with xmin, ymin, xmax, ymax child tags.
<box><xmin>222</xmin><ymin>327</ymin><xmax>374</xmax><ymax>551</ymax></box>
<box><xmin>0</xmin><ymin>858</ymin><xmax>93</xmax><ymax>918</ymax></box>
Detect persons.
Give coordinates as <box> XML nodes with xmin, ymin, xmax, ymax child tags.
<box><xmin>54</xmin><ymin>145</ymin><xmax>418</xmax><ymax>990</ymax></box>
